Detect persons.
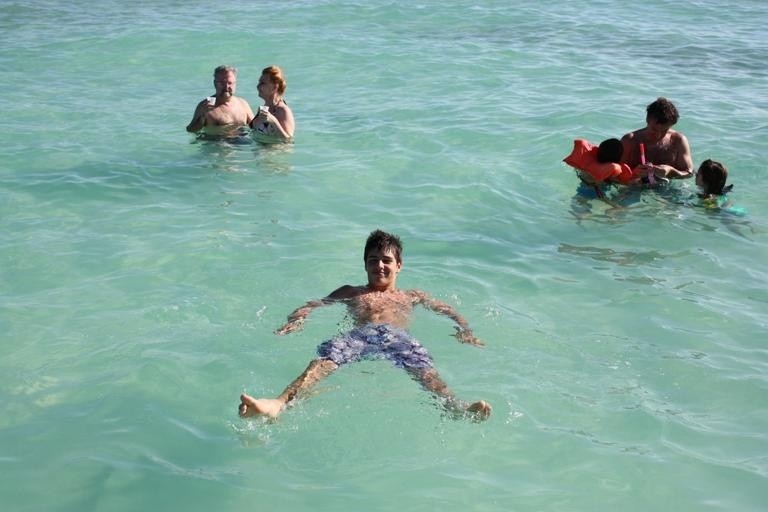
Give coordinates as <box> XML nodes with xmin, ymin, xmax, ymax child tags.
<box><xmin>695</xmin><ymin>158</ymin><xmax>746</xmax><ymax>216</ymax></box>
<box><xmin>620</xmin><ymin>98</ymin><xmax>695</xmax><ymax>186</ymax></box>
<box><xmin>186</xmin><ymin>65</ymin><xmax>255</xmax><ymax>132</ymax></box>
<box><xmin>577</xmin><ymin>138</ymin><xmax>625</xmax><ymax>200</ymax></box>
<box><xmin>238</xmin><ymin>230</ymin><xmax>491</xmax><ymax>423</ymax></box>
<box><xmin>248</xmin><ymin>65</ymin><xmax>291</xmax><ymax>140</ymax></box>
